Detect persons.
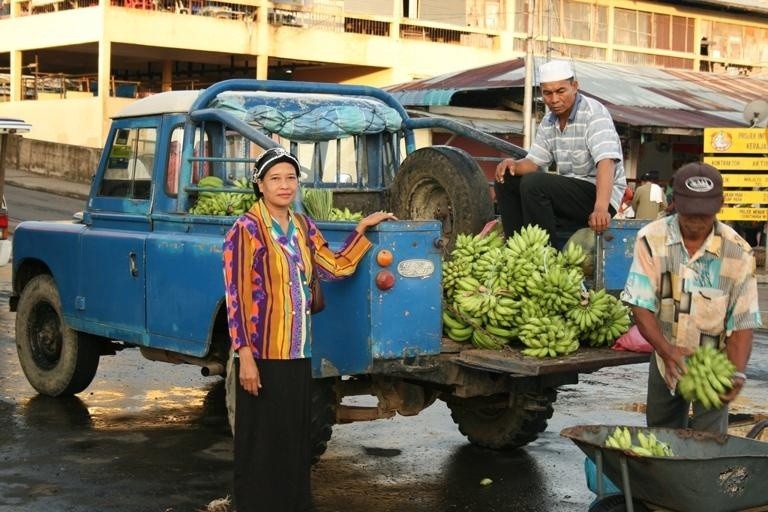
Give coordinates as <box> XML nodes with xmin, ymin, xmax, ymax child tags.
<box><xmin>632</xmin><ymin>171</ymin><xmax>668</xmax><ymax>220</ymax></box>
<box><xmin>665</xmin><ymin>173</ymin><xmax>678</xmax><ymax>217</ymax></box>
<box><xmin>620</xmin><ymin>163</ymin><xmax>762</xmax><ymax>436</ymax></box>
<box><xmin>493</xmin><ymin>59</ymin><xmax>627</xmax><ymax>254</ymax></box>
<box><xmin>220</xmin><ymin>148</ymin><xmax>399</xmax><ymax>512</ymax></box>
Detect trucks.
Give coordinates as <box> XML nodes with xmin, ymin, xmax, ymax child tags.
<box><xmin>8</xmin><ymin>75</ymin><xmax>655</xmax><ymax>451</ymax></box>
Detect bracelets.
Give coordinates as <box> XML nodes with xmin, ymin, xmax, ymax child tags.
<box><xmin>732</xmin><ymin>372</ymin><xmax>747</xmax><ymax>381</ymax></box>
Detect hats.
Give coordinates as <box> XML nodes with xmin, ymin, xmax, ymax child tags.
<box><xmin>538</xmin><ymin>59</ymin><xmax>577</xmax><ymax>82</ymax></box>
<box><xmin>672</xmin><ymin>163</ymin><xmax>724</xmax><ymax>215</ymax></box>
<box><xmin>253</xmin><ymin>147</ymin><xmax>299</xmax><ymax>198</ymax></box>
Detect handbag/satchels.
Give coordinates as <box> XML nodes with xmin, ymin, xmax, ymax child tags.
<box><xmin>311</xmin><ymin>279</ymin><xmax>325</xmax><ymax>314</ymax></box>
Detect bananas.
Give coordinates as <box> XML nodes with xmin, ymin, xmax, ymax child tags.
<box><xmin>190</xmin><ymin>177</ymin><xmax>364</xmax><ymax>225</ymax></box>
<box><xmin>606</xmin><ymin>427</ymin><xmax>676</xmax><ymax>459</ymax></box>
<box><xmin>441</xmin><ymin>223</ymin><xmax>633</xmax><ymax>358</ymax></box>
<box><xmin>679</xmin><ymin>342</ymin><xmax>737</xmax><ymax>410</ymax></box>
